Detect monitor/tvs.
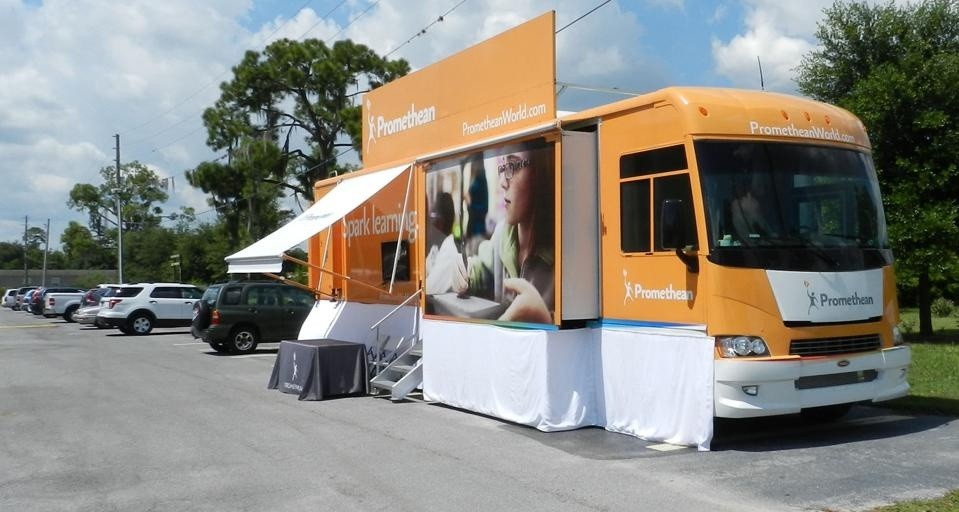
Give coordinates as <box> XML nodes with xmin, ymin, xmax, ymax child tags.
<box><xmin>380</xmin><ymin>240</ymin><xmax>412</xmax><ymax>281</ymax></box>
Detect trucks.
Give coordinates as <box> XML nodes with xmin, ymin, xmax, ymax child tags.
<box><xmin>220</xmin><ymin>9</ymin><xmax>912</xmax><ymax>448</ymax></box>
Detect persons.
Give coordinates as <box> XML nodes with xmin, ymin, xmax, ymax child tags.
<box><xmin>448</xmin><ymin>138</ymin><xmax>555</xmax><ymax>325</ymax></box>
<box><xmin>729</xmin><ymin>170</ymin><xmax>791</xmax><ymax>248</ymax></box>
<box><xmin>458</xmin><ymin>154</ymin><xmax>489</xmax><ymax>243</ymax></box>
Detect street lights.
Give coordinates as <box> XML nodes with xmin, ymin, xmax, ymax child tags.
<box><xmin>110</xmin><ymin>132</ymin><xmax>126</xmax><ymax>283</ymax></box>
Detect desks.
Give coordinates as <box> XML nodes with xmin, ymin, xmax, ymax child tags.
<box><xmin>279</xmin><ymin>338</ymin><xmax>364</xmax><ymax>398</ymax></box>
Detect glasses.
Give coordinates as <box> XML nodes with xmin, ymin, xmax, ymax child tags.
<box><xmin>497</xmin><ymin>158</ymin><xmax>535</xmax><ymax>179</ymax></box>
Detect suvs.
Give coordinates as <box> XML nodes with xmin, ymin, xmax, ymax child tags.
<box><xmin>2</xmin><ymin>282</ymin><xmax>204</xmax><ymax>336</ymax></box>
<box><xmin>190</xmin><ymin>281</ymin><xmax>319</xmax><ymax>357</ymax></box>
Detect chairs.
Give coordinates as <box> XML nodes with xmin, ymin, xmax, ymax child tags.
<box><xmin>659</xmin><ymin>196</ymin><xmax>697</xmax><ymax>249</ymax></box>
<box><xmin>255</xmin><ymin>294</ymin><xmax>276</xmax><ymax>305</ymax></box>
